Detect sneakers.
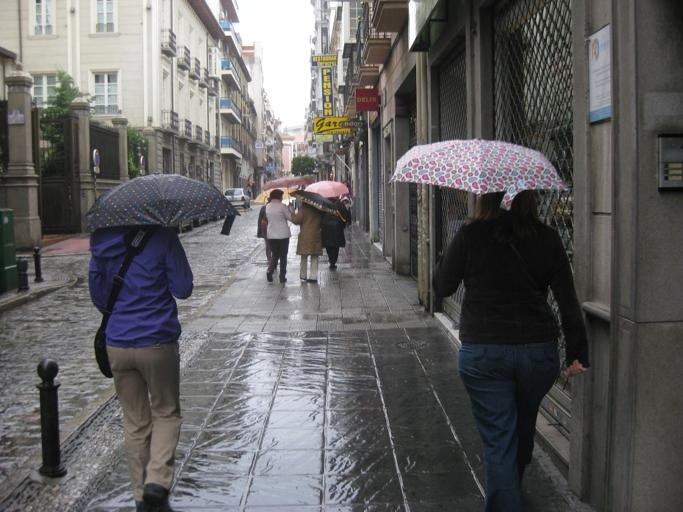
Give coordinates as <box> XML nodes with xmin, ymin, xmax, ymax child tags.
<box><xmin>136</xmin><ymin>483</ymin><xmax>174</xmax><ymax>512</ymax></box>
<box><xmin>299</xmin><ymin>276</ymin><xmax>318</xmax><ymax>284</ymax></box>
<box><xmin>280</xmin><ymin>277</ymin><xmax>287</xmax><ymax>283</ymax></box>
<box><xmin>266</xmin><ymin>271</ymin><xmax>273</xmax><ymax>282</ymax></box>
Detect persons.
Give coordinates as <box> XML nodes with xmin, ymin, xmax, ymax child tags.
<box><xmin>433</xmin><ymin>189</ymin><xmax>589</xmax><ymax>510</ymax></box>
<box><xmin>89</xmin><ymin>226</ymin><xmax>197</xmax><ymax>512</ymax></box>
<box><xmin>255</xmin><ymin>182</ymin><xmax>353</xmax><ymax>282</ymax></box>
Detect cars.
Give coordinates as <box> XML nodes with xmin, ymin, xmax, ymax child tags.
<box><xmin>224</xmin><ymin>188</ymin><xmax>250</xmax><ymax>209</ymax></box>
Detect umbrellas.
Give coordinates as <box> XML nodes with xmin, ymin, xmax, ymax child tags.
<box><xmin>304</xmin><ymin>180</ymin><xmax>349</xmax><ymax>196</ymax></box>
<box><xmin>254</xmin><ymin>187</ymin><xmax>297</xmax><ymax>204</ymax></box>
<box><xmin>84</xmin><ymin>170</ymin><xmax>240</xmax><ymax>229</ymax></box>
<box><xmin>263</xmin><ymin>175</ymin><xmax>314</xmax><ymax>189</ymax></box>
<box><xmin>389</xmin><ymin>137</ymin><xmax>569</xmax><ymax>210</ymax></box>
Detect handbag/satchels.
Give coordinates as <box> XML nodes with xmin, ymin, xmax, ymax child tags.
<box><xmin>94</xmin><ymin>327</ymin><xmax>114</xmax><ymax>378</ymax></box>
